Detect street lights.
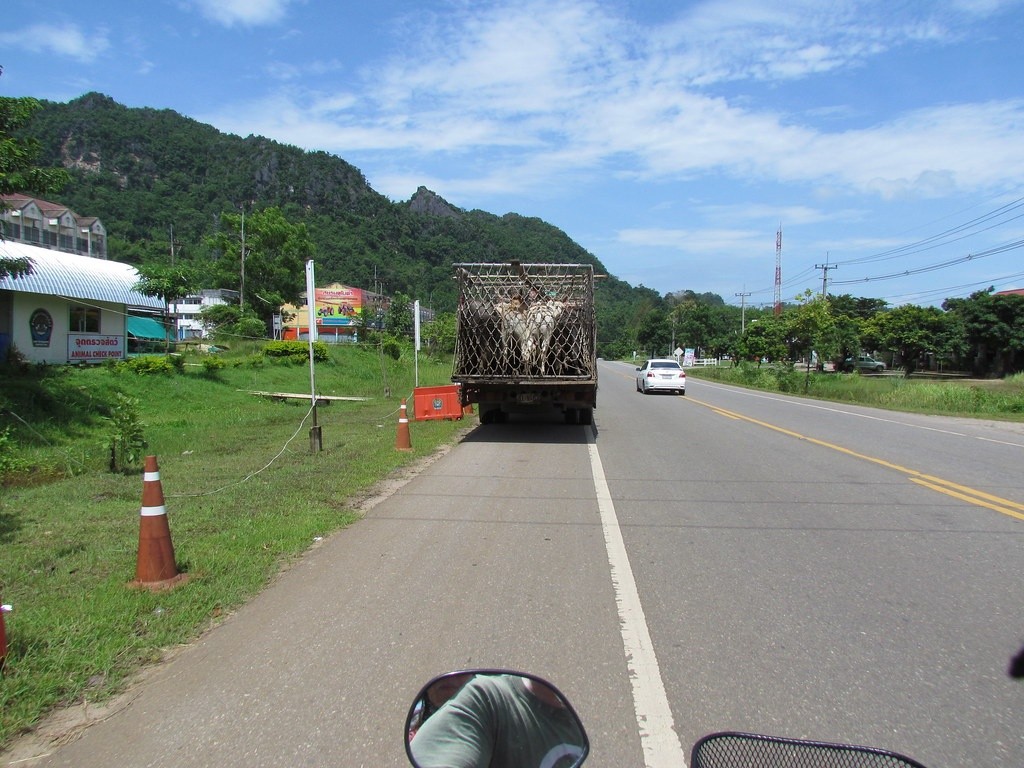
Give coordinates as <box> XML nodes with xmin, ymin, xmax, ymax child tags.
<box><xmin>228</xmin><ymin>232</ymin><xmax>246</xmax><ymax>332</ymax></box>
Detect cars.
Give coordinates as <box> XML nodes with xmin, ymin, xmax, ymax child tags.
<box><xmin>636</xmin><ymin>359</ymin><xmax>687</xmax><ymax>396</ymax></box>
<box><xmin>338</xmin><ymin>302</ymin><xmax>354</xmax><ymax>316</ymax></box>
<box><xmin>318</xmin><ymin>306</ymin><xmax>334</xmax><ymax>317</ymax></box>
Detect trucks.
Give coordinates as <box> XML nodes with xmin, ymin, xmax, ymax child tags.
<box><xmin>450</xmin><ymin>260</ymin><xmax>610</xmax><ymax>426</ymax></box>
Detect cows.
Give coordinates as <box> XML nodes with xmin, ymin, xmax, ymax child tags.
<box><xmin>455</xmin><ymin>284</ymin><xmax>589</xmax><ymax>377</ymax></box>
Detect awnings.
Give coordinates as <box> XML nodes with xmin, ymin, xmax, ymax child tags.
<box><xmin>126</xmin><ymin>315</ymin><xmax>175</xmax><ymax>342</ymax></box>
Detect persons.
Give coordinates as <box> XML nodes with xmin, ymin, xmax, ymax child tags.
<box><xmin>408</xmin><ymin>673</ymin><xmax>581</xmax><ymax>768</ymax></box>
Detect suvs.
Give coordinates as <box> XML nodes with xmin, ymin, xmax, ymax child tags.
<box><xmin>833</xmin><ymin>355</ymin><xmax>887</xmax><ymax>374</ymax></box>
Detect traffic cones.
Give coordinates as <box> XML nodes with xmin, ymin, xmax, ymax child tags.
<box><xmin>390</xmin><ymin>398</ymin><xmax>413</xmax><ymax>452</ymax></box>
<box><xmin>125</xmin><ymin>455</ymin><xmax>191</xmax><ymax>592</ymax></box>
<box><xmin>464</xmin><ymin>395</ymin><xmax>473</xmax><ymax>417</ymax></box>
